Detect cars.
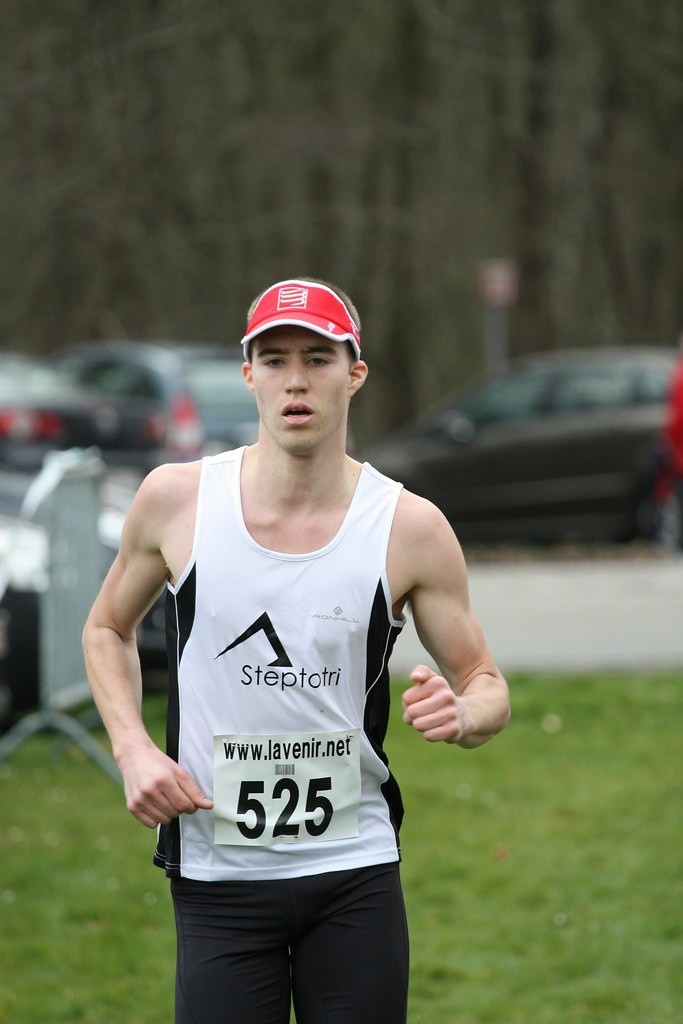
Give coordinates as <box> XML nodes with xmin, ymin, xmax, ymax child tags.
<box><xmin>0</xmin><ymin>338</ymin><xmax>262</xmax><ymax>735</ymax></box>
<box><xmin>367</xmin><ymin>339</ymin><xmax>682</xmax><ymax>557</ymax></box>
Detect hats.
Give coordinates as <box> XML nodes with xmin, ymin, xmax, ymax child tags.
<box><xmin>240</xmin><ymin>279</ymin><xmax>360</xmax><ymax>363</ymax></box>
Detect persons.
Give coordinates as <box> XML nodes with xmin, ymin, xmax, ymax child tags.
<box><xmin>81</xmin><ymin>275</ymin><xmax>509</xmax><ymax>1024</ymax></box>
